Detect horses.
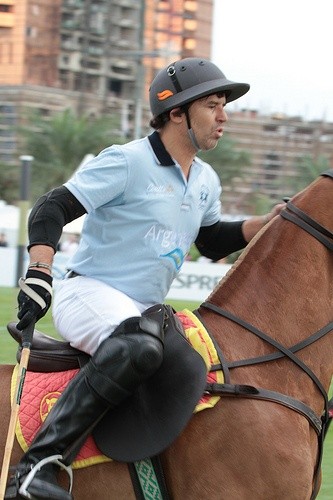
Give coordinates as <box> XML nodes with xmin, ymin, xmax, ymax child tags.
<box><xmin>0</xmin><ymin>169</ymin><xmax>333</xmax><ymax>500</ymax></box>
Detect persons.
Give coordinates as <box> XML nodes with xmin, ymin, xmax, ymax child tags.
<box><xmin>16</xmin><ymin>56</ymin><xmax>289</xmax><ymax>500</ymax></box>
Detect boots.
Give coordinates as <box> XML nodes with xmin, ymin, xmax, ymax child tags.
<box><xmin>7</xmin><ymin>317</ymin><xmax>163</xmax><ymax>498</ymax></box>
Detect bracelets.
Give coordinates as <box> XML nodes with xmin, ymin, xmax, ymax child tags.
<box><xmin>28</xmin><ymin>262</ymin><xmax>52</xmax><ymax>271</ymax></box>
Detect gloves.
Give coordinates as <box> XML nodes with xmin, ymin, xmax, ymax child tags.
<box><xmin>16</xmin><ymin>269</ymin><xmax>54</xmax><ymax>330</ymax></box>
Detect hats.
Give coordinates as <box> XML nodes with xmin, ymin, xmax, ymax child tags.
<box><xmin>149</xmin><ymin>58</ymin><xmax>249</xmax><ymax>117</ymax></box>
